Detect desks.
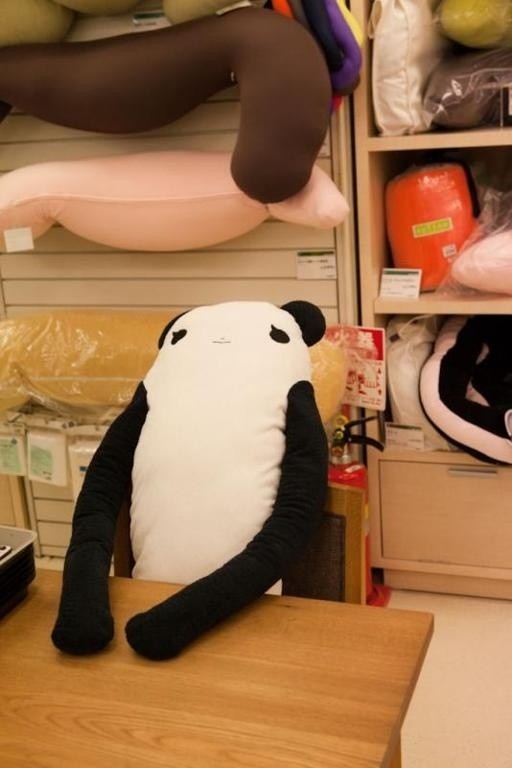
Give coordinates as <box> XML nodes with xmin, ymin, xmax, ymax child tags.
<box><xmin>0</xmin><ymin>569</ymin><xmax>434</xmax><ymax>768</ymax></box>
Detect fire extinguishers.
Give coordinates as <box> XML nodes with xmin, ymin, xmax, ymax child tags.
<box><xmin>326</xmin><ymin>416</ymin><xmax>384</xmax><ymax>597</ymax></box>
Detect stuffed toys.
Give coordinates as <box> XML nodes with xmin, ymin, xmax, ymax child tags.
<box><xmin>48</xmin><ymin>298</ymin><xmax>327</xmax><ymax>658</ymax></box>
